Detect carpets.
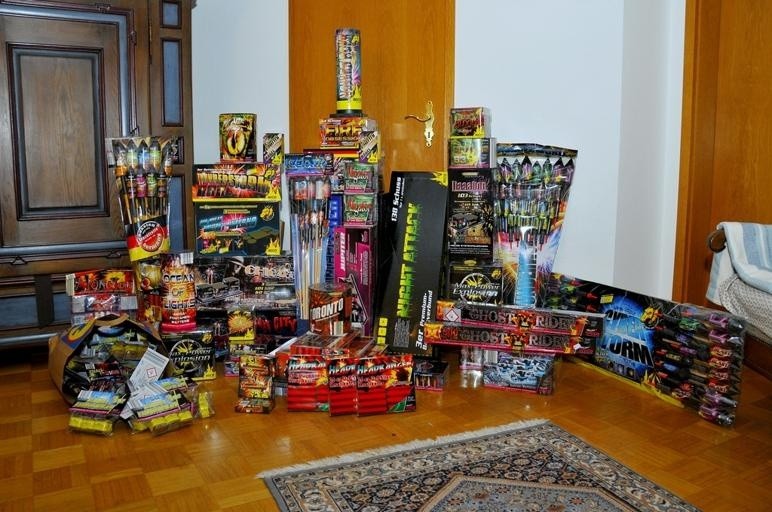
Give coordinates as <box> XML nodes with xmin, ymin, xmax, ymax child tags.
<box><xmin>253</xmin><ymin>418</ymin><xmax>703</xmax><ymax>512</ymax></box>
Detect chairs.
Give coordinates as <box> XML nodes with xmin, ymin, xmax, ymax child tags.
<box><xmin>705</xmin><ymin>221</ymin><xmax>772</xmax><ymax>381</ymax></box>
